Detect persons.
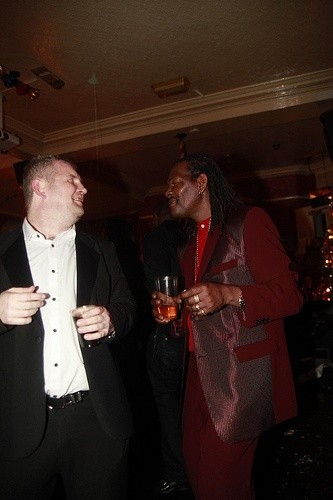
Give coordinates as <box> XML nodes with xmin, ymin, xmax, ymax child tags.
<box><xmin>0</xmin><ymin>151</ymin><xmax>313</xmax><ymax>500</ymax></box>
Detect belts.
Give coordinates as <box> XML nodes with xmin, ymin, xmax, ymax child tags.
<box><xmin>46</xmin><ymin>391</ymin><xmax>89</xmax><ymax>409</ymax></box>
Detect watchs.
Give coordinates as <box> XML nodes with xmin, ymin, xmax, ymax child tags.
<box><xmin>239</xmin><ymin>291</ymin><xmax>245</xmax><ymax>308</ymax></box>
<box><xmin>101</xmin><ymin>330</ymin><xmax>115</xmax><ymax>344</ymax></box>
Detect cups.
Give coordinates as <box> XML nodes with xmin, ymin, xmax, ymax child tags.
<box><xmin>72</xmin><ymin>305</ymin><xmax>102</xmax><ymax>351</ymax></box>
<box><xmin>155</xmin><ymin>275</ymin><xmax>179</xmax><ymax>319</ymax></box>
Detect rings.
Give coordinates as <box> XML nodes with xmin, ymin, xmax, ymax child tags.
<box><xmin>196</xmin><ymin>304</ymin><xmax>200</xmax><ymax>311</ymax></box>
<box><xmin>193</xmin><ymin>295</ymin><xmax>200</xmax><ymax>303</ymax></box>
<box><xmin>201</xmin><ymin>310</ymin><xmax>206</xmax><ymax>316</ymax></box>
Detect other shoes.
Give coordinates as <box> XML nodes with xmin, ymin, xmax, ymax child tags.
<box><xmin>144</xmin><ymin>471</ymin><xmax>192</xmax><ymax>495</ymax></box>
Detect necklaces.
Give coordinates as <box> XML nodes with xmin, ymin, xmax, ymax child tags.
<box><xmin>196</xmin><ymin>217</ymin><xmax>211</xmax><ymax>267</ymax></box>
